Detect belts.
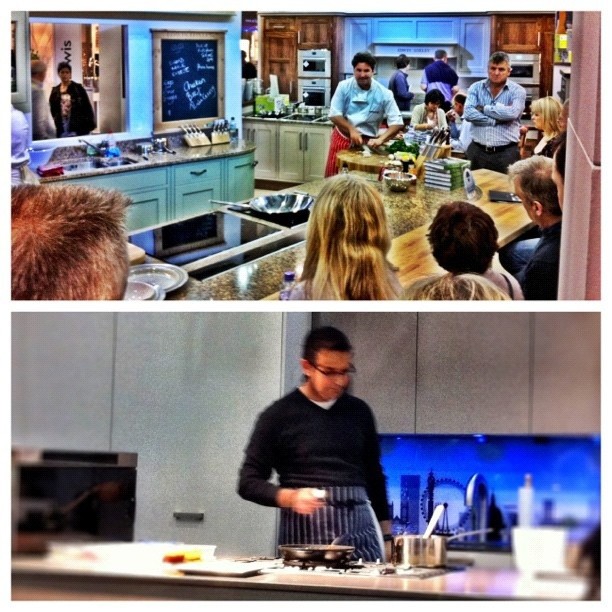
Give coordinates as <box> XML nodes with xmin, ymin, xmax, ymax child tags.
<box><xmin>474</xmin><ymin>142</ymin><xmax>518</xmax><ymax>152</ymax></box>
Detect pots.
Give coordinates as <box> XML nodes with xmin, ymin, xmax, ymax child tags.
<box><xmin>135</xmin><ymin>142</ymin><xmax>154</xmax><ymax>161</ymax></box>
<box><xmin>152</xmin><ymin>137</ymin><xmax>177</xmax><ymax>155</ymax></box>
<box><xmin>392</xmin><ymin>528</ymin><xmax>491</xmax><ymax>566</ymax></box>
<box><xmin>210</xmin><ymin>191</ymin><xmax>314</xmax><ymax>212</ymax></box>
<box><xmin>277</xmin><ymin>533</ymin><xmax>353</xmax><ymax>564</ymax></box>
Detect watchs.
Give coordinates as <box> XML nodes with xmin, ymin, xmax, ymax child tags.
<box><xmin>383</xmin><ymin>535</ymin><xmax>394</xmax><ymax>541</ymax></box>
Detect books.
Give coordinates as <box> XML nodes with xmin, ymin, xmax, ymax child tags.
<box><xmin>424</xmin><ymin>157</ymin><xmax>472</xmax><ymax>191</ymax></box>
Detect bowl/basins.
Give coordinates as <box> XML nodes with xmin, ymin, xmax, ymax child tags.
<box><xmin>384</xmin><ymin>170</ymin><xmax>414</xmax><ymax>191</ymax></box>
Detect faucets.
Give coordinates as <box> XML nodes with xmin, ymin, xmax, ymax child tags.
<box><xmin>465</xmin><ymin>472</ymin><xmax>488</xmax><ymax>542</ymax></box>
<box><xmin>292</xmin><ymin>101</ymin><xmax>306</xmax><ymax>112</ymax></box>
<box><xmin>79</xmin><ymin>140</ymin><xmax>107</xmax><ymax>157</ymax></box>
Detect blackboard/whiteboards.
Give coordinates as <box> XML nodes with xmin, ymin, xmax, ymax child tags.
<box><xmin>149</xmin><ymin>29</ymin><xmax>228</xmax><ymax>136</ymax></box>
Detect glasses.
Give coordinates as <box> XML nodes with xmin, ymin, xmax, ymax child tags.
<box><xmin>310</xmin><ymin>362</ymin><xmax>358</xmax><ymax>379</ymax></box>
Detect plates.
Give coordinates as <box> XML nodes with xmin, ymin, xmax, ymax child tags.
<box><xmin>122</xmin><ymin>263</ymin><xmax>187</xmax><ymax>300</ymax></box>
<box><xmin>179</xmin><ymin>559</ymin><xmax>262</xmax><ymax>575</ymax></box>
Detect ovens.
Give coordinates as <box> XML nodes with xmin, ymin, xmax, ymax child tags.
<box><xmin>11</xmin><ymin>451</ymin><xmax>139</xmax><ymax>551</ymax></box>
<box><xmin>299</xmin><ymin>48</ymin><xmax>331</xmax><ymax>108</ymax></box>
<box><xmin>509</xmin><ymin>53</ymin><xmax>543</xmax><ymax>120</ymax></box>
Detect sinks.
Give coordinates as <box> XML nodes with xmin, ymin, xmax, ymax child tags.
<box><xmin>107</xmin><ymin>160</ymin><xmax>139</xmax><ymax>166</ymax></box>
<box><xmin>284</xmin><ymin>114</ymin><xmax>322</xmax><ymax>121</ymax></box>
<box><xmin>316</xmin><ymin>117</ymin><xmax>329</xmax><ymax>122</ymax></box>
<box><xmin>64</xmin><ymin>161</ymin><xmax>107</xmax><ymax>171</ymax></box>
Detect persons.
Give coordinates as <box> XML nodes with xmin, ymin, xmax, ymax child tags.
<box><xmin>388</xmin><ymin>54</ymin><xmax>414</xmax><ymax>110</ymax></box>
<box><xmin>30</xmin><ymin>60</ymin><xmax>53</xmax><ymax>140</ymax></box>
<box><xmin>12</xmin><ymin>183</ymin><xmax>132</xmax><ymax>300</ymax></box>
<box><xmin>325</xmin><ymin>51</ymin><xmax>404</xmax><ymax>177</ymax></box>
<box><xmin>408</xmin><ymin>89</ymin><xmax>449</xmax><ymax>148</ymax></box>
<box><xmin>463</xmin><ymin>52</ymin><xmax>527</xmax><ymax>174</ymax></box>
<box><xmin>49</xmin><ymin>63</ymin><xmax>96</xmax><ymax>135</ymax></box>
<box><xmin>420</xmin><ymin>49</ymin><xmax>459</xmax><ymax>103</ymax></box>
<box><xmin>527</xmin><ymin>98</ymin><xmax>562</xmax><ymax>155</ymax></box>
<box><xmin>428</xmin><ymin>201</ymin><xmax>523</xmax><ymax>301</ymax></box>
<box><xmin>289</xmin><ymin>173</ymin><xmax>402</xmax><ymax>299</ymax></box>
<box><xmin>509</xmin><ymin>156</ymin><xmax>556</xmax><ymax>300</ymax></box>
<box><xmin>237</xmin><ymin>327</ymin><xmax>397</xmax><ymax>562</ymax></box>
<box><xmin>446</xmin><ymin>94</ymin><xmax>466</xmax><ymax>147</ymax></box>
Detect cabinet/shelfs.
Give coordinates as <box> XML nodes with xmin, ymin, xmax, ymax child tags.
<box><xmin>10</xmin><ymin>314</ymin><xmax>115</xmax><ymax>451</ymax></box>
<box><xmin>113</xmin><ymin>313</ymin><xmax>283</xmax><ymax>557</ymax></box>
<box><xmin>531</xmin><ymin>312</ymin><xmax>601</xmax><ymax>435</ymax></box>
<box><xmin>499</xmin><ymin>15</ymin><xmax>543</xmax><ymax>54</ymax></box>
<box><xmin>456</xmin><ymin>17</ymin><xmax>491</xmax><ymax>78</ymax></box>
<box><xmin>278</xmin><ymin>123</ymin><xmax>331</xmax><ymax>183</ymax></box>
<box><xmin>314</xmin><ymin>312</ymin><xmax>415</xmax><ymax>437</ymax></box>
<box><xmin>415</xmin><ymin>313</ymin><xmax>533</xmax><ymax>436</ymax></box>
<box><xmin>225</xmin><ymin>152</ymin><xmax>258</xmax><ymax>207</ymax></box>
<box><xmin>258</xmin><ymin>15</ymin><xmax>297</xmax><ymax>101</ymax></box>
<box><xmin>343</xmin><ymin>19</ymin><xmax>374</xmax><ymax>73</ymax></box>
<box><xmin>41</xmin><ymin>168</ymin><xmax>171</xmax><ymax>229</ymax></box>
<box><xmin>298</xmin><ymin>16</ymin><xmax>334</xmax><ymax>51</ymax></box>
<box><xmin>244</xmin><ymin>121</ymin><xmax>277</xmax><ymax>186</ymax></box>
<box><xmin>174</xmin><ymin>161</ymin><xmax>226</xmax><ymax>222</ymax></box>
